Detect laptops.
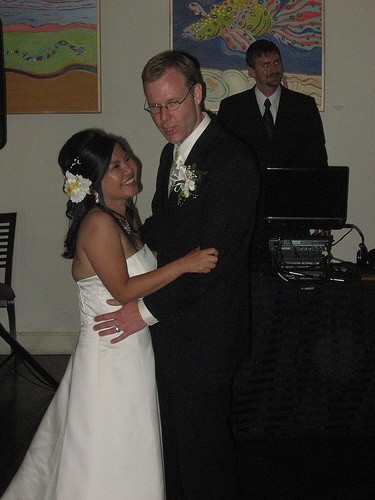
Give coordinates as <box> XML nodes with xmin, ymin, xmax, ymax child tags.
<box><xmin>263</xmin><ymin>165</ymin><xmax>348</xmax><ymax>229</ymax></box>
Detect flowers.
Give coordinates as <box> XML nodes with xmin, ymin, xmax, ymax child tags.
<box><xmin>171</xmin><ymin>158</ymin><xmax>208</xmax><ymax>206</ymax></box>
<box><xmin>65</xmin><ymin>170</ymin><xmax>94</xmax><ymax>204</ymax></box>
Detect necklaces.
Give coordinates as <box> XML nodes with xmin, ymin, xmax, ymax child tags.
<box><xmin>119</xmin><ymin>217</ymin><xmax>131</xmax><ymax>237</ymax></box>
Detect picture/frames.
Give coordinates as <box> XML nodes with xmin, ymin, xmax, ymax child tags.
<box><xmin>168</xmin><ymin>0</ymin><xmax>325</xmax><ymax>111</ymax></box>
<box><xmin>0</xmin><ymin>0</ymin><xmax>101</xmax><ymax>115</ymax></box>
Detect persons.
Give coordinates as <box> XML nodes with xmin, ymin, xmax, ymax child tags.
<box><xmin>57</xmin><ymin>130</ymin><xmax>218</xmax><ymax>500</ymax></box>
<box><xmin>92</xmin><ymin>51</ymin><xmax>259</xmax><ymax>499</ymax></box>
<box><xmin>217</xmin><ymin>39</ymin><xmax>328</xmax><ymax>240</ymax></box>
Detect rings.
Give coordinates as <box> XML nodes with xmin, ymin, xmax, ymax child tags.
<box><xmin>114</xmin><ymin>326</ymin><xmax>119</xmax><ymax>332</ymax></box>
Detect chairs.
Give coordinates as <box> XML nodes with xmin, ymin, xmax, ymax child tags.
<box><xmin>0</xmin><ymin>211</ymin><xmax>17</xmax><ymax>374</ymax></box>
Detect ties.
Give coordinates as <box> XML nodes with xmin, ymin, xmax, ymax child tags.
<box><xmin>262</xmin><ymin>98</ymin><xmax>275</xmax><ymax>129</ymax></box>
<box><xmin>167</xmin><ymin>148</ymin><xmax>185</xmax><ymax>199</ymax></box>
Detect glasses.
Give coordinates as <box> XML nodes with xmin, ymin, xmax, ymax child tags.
<box><xmin>143</xmin><ymin>81</ymin><xmax>196</xmax><ymax>114</ymax></box>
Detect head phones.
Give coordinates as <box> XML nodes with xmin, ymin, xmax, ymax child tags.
<box><xmin>356</xmin><ymin>243</ymin><xmax>375</xmax><ymax>269</ymax></box>
